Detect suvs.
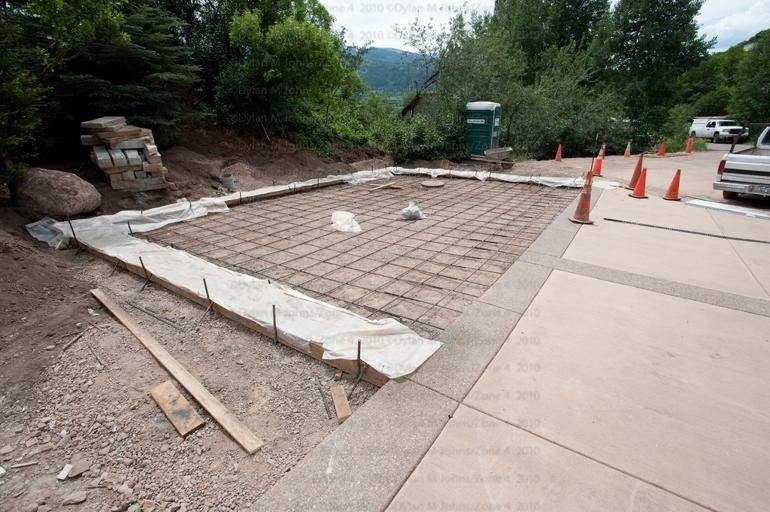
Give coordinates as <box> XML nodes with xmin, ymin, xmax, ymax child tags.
<box><xmin>689</xmin><ymin>117</ymin><xmax>749</xmax><ymax>143</ymax></box>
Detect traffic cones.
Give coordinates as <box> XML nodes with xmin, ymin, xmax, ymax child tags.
<box><xmin>628</xmin><ymin>167</ymin><xmax>648</xmax><ymax>198</ymax></box>
<box><xmin>662</xmin><ymin>168</ymin><xmax>682</xmax><ymax>201</ymax></box>
<box><xmin>625</xmin><ymin>153</ymin><xmax>644</xmax><ymax>190</ymax></box>
<box><xmin>591</xmin><ymin>159</ymin><xmax>606</xmax><ymax>175</ymax></box>
<box><xmin>567</xmin><ymin>170</ymin><xmax>594</xmax><ymax>224</ymax></box>
<box><xmin>596</xmin><ymin>138</ymin><xmax>694</xmax><ymax>160</ymax></box>
<box><xmin>555</xmin><ymin>143</ymin><xmax>562</xmax><ymax>161</ymax></box>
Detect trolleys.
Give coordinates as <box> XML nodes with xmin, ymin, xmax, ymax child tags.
<box><xmin>470</xmin><ymin>146</ymin><xmax>516</xmax><ymax>169</ymax></box>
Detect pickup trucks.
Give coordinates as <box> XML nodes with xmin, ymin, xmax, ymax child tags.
<box><xmin>713</xmin><ymin>125</ymin><xmax>770</xmax><ymax>202</ymax></box>
